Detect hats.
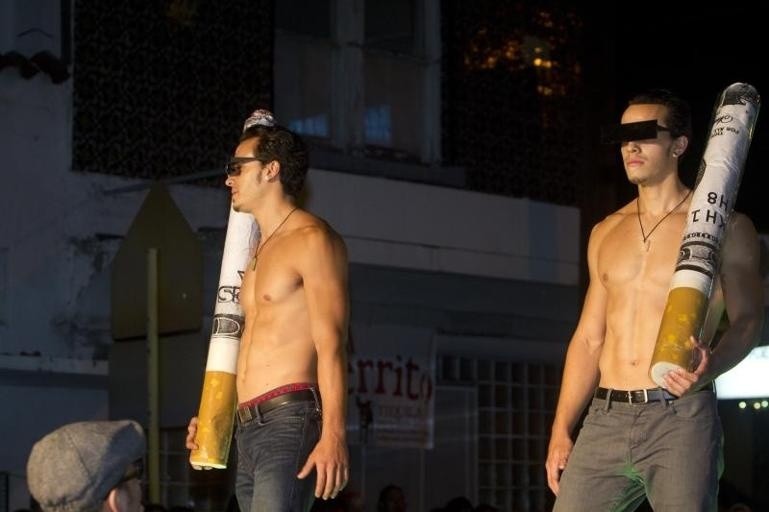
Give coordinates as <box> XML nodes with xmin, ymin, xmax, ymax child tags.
<box><xmin>25</xmin><ymin>420</ymin><xmax>146</xmax><ymax>511</ymax></box>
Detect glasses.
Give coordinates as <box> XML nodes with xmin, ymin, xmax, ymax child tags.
<box><xmin>225</xmin><ymin>158</ymin><xmax>260</xmax><ymax>176</ymax></box>
<box><xmin>612</xmin><ymin>120</ymin><xmax>674</xmax><ymax>144</ymax></box>
<box><xmin>105</xmin><ymin>463</ymin><xmax>145</xmax><ymax>497</ymax></box>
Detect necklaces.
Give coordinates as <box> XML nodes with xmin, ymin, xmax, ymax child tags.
<box><xmin>633</xmin><ymin>188</ymin><xmax>693</xmax><ymax>252</ymax></box>
<box><xmin>247</xmin><ymin>207</ymin><xmax>297</xmax><ymax>273</ymax></box>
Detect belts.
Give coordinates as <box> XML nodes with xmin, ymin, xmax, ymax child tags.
<box><xmin>592</xmin><ymin>383</ymin><xmax>717</xmax><ymax>401</ymax></box>
<box><xmin>234</xmin><ymin>389</ymin><xmax>322</xmax><ymax>422</ymax></box>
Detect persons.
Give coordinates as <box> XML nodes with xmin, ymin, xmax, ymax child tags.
<box><xmin>185</xmin><ymin>122</ymin><xmax>350</xmax><ymax>511</ymax></box>
<box><xmin>22</xmin><ymin>415</ymin><xmax>157</xmax><ymax>510</ymax></box>
<box><xmin>544</xmin><ymin>96</ymin><xmax>768</xmax><ymax>512</ymax></box>
<box><xmin>378</xmin><ymin>484</ymin><xmax>406</xmax><ymax>511</ymax></box>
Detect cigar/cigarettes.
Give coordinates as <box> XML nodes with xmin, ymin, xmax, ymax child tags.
<box><xmin>189</xmin><ymin>108</ymin><xmax>275</xmax><ymax>474</ymax></box>
<box><xmin>646</xmin><ymin>82</ymin><xmax>760</xmax><ymax>392</ymax></box>
